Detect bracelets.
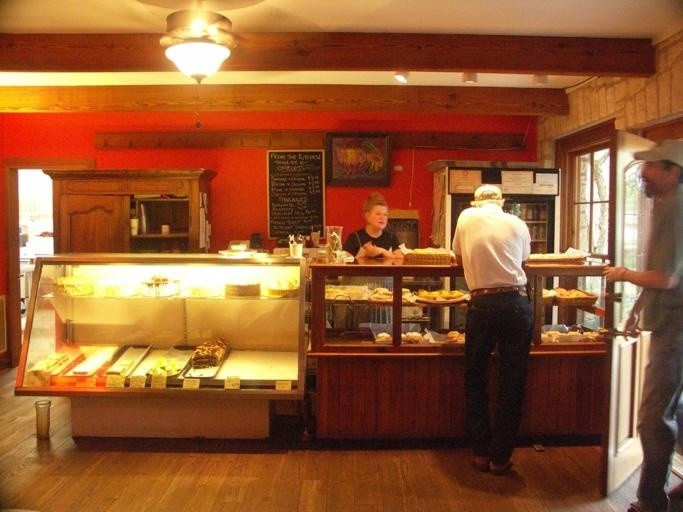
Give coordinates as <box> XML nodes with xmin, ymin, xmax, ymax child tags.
<box><xmin>619</xmin><ymin>268</ymin><xmax>629</xmax><ymax>282</ymax></box>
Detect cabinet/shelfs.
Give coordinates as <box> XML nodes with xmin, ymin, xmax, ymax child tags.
<box><xmin>505</xmin><ymin>202</ymin><xmax>548</xmax><ymax>253</ymax></box>
<box><xmin>40</xmin><ymin>165</ymin><xmax>216</xmax><ymax>254</ymax></box>
<box><xmin>303</xmin><ymin>246</ymin><xmax>611</xmax><ymax>447</ymax></box>
<box><xmin>12</xmin><ymin>252</ymin><xmax>305</xmax><ymax>441</ymax></box>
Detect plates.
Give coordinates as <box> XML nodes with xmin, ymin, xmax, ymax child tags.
<box><xmin>285</xmin><ymin>255</ymin><xmax>307</xmax><ymax>261</ymax></box>
<box><xmin>216</xmin><ymin>248</ymin><xmax>258</xmax><ymax>259</ymax></box>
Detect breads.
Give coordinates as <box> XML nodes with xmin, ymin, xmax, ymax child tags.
<box><xmin>370</xmin><ymin>284</ymin><xmax>465</xmax><ymax>343</ymax></box>
<box><xmin>541</xmin><ymin>287</ymin><xmax>600</xmax><ymax>342</ymax></box>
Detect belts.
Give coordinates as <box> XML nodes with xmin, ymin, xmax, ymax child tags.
<box><xmin>470</xmin><ymin>287</ymin><xmax>526</xmax><ymax>296</ymax></box>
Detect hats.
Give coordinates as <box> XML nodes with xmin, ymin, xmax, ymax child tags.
<box><xmin>631</xmin><ymin>139</ymin><xmax>682</xmax><ymax>167</ymax></box>
<box><xmin>470</xmin><ymin>184</ymin><xmax>505</xmax><ymax>208</ymax></box>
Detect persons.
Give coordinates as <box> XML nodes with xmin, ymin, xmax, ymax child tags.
<box><xmin>449</xmin><ymin>183</ymin><xmax>536</xmax><ymax>477</ymax></box>
<box><xmin>596</xmin><ymin>136</ymin><xmax>681</xmax><ymax>511</ymax></box>
<box><xmin>342</xmin><ymin>192</ymin><xmax>406</xmax><ymax>262</ymax></box>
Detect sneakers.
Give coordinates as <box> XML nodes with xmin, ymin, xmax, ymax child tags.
<box><xmin>627</xmin><ymin>501</ymin><xmax>645</xmax><ymax>512</ymax></box>
<box><xmin>668</xmin><ymin>479</ymin><xmax>682</xmax><ymax>500</ymax></box>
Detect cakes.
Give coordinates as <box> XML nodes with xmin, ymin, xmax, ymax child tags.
<box><xmin>141</xmin><ymin>275</ymin><xmax>178</xmax><ymax>297</ymax></box>
<box><xmin>52</xmin><ymin>276</ymin><xmax>94</xmax><ymax>297</ymax></box>
<box><xmin>191</xmin><ymin>335</ymin><xmax>229</xmax><ymax>369</ymax></box>
<box><xmin>268</xmin><ymin>279</ymin><xmax>299</xmax><ymax>297</ymax></box>
<box><xmin>224</xmin><ymin>282</ymin><xmax>261</xmax><ymax>297</ymax></box>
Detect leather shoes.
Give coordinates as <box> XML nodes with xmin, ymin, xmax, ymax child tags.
<box><xmin>474</xmin><ymin>454</ymin><xmax>489</xmax><ymax>469</ymax></box>
<box><xmin>489</xmin><ymin>457</ymin><xmax>512</xmax><ymax>474</ymax></box>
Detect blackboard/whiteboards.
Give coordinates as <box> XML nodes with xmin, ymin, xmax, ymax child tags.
<box><xmin>266</xmin><ymin>149</ymin><xmax>326</xmax><ymax>240</ymax></box>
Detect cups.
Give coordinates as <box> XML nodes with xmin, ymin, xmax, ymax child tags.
<box><xmin>129</xmin><ymin>219</ymin><xmax>138</xmax><ymax>235</ymax></box>
<box><xmin>311</xmin><ymin>224</ymin><xmax>321</xmax><ymax>248</ymax></box>
<box><xmin>289</xmin><ymin>244</ymin><xmax>304</xmax><ymax>258</ymax></box>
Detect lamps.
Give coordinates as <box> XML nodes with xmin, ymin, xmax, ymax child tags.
<box><xmin>157</xmin><ymin>24</ymin><xmax>240</xmax><ymax>87</ymax></box>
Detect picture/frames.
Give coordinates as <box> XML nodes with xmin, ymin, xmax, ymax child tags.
<box><xmin>325</xmin><ymin>131</ymin><xmax>392</xmax><ymax>187</ymax></box>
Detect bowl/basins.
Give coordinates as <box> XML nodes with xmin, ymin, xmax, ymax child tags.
<box><xmin>229</xmin><ymin>244</ymin><xmax>249</xmax><ymax>252</ymax></box>
